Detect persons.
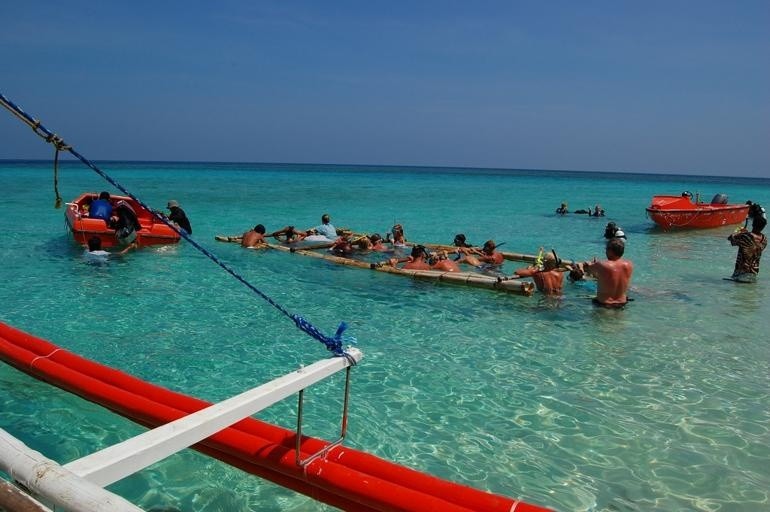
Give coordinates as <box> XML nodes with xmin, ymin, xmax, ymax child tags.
<box><xmin>329</xmin><ymin>225</ymin><xmax>404</xmax><ymax>251</ymax></box>
<box><xmin>454</xmin><ymin>234</ymin><xmax>472</xmax><ymax>247</ymax></box>
<box><xmin>477</xmin><ymin>240</ymin><xmax>504</xmax><ymax>264</ymax></box>
<box><xmin>166</xmin><ymin>200</ymin><xmax>192</xmax><ymax>237</ymax></box>
<box><xmin>90</xmin><ymin>191</ymin><xmax>113</xmax><ymax>221</ymax></box>
<box><xmin>401</xmin><ymin>243</ymin><xmax>459</xmax><ymax>272</ymax></box>
<box><xmin>110</xmin><ymin>199</ymin><xmax>137</xmax><ymax>247</ymax></box>
<box><xmin>556</xmin><ymin>201</ymin><xmax>568</xmax><ymax>214</ymax></box>
<box><xmin>584</xmin><ymin>240</ymin><xmax>632</xmax><ymax>308</ymax></box>
<box><xmin>515</xmin><ymin>252</ymin><xmax>563</xmax><ymax>293</ymax></box>
<box><xmin>85</xmin><ymin>236</ymin><xmax>134</xmax><ymax>267</ymax></box>
<box><xmin>608</xmin><ymin>222</ymin><xmax>627</xmax><ymax>240</ymax></box>
<box><xmin>314</xmin><ymin>215</ymin><xmax>337</xmax><ymax>239</ymax></box>
<box><xmin>728</xmin><ymin>216</ymin><xmax>768</xmax><ymax>283</ymax></box>
<box><xmin>274</xmin><ymin>227</ymin><xmax>307</xmax><ymax>242</ymax></box>
<box><xmin>746</xmin><ymin>200</ymin><xmax>765</xmax><ymax>219</ymax></box>
<box><xmin>242</xmin><ymin>224</ymin><xmax>266</xmax><ymax>246</ymax></box>
<box><xmin>588</xmin><ymin>205</ymin><xmax>607</xmax><ymax>216</ymax></box>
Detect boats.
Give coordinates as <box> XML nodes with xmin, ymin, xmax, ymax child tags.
<box><xmin>64</xmin><ymin>192</ymin><xmax>181</xmax><ymax>247</ymax></box>
<box><xmin>646</xmin><ymin>195</ymin><xmax>753</xmax><ymax>231</ymax></box>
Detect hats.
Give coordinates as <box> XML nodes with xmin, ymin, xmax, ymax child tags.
<box><xmin>166</xmin><ymin>200</ymin><xmax>178</xmax><ymax>208</ymax></box>
